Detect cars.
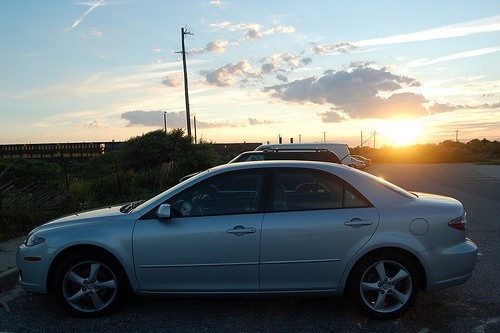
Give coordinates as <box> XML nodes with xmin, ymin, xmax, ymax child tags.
<box><xmin>15</xmin><ymin>159</ymin><xmax>477</xmax><ymax>319</ymax></box>
<box><xmin>351</xmin><ymin>154</ymin><xmax>371</xmax><ymax>167</ymax></box>
<box><xmin>350</xmin><ymin>157</ymin><xmax>364</xmax><ymax>169</ymax></box>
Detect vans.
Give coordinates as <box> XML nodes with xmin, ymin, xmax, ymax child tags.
<box><xmin>253</xmin><ymin>142</ymin><xmax>352</xmax><ymax>167</ymax></box>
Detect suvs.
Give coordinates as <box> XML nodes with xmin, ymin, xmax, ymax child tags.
<box><xmin>180</xmin><ymin>148</ymin><xmax>344</xmax><ymax>212</ymax></box>
